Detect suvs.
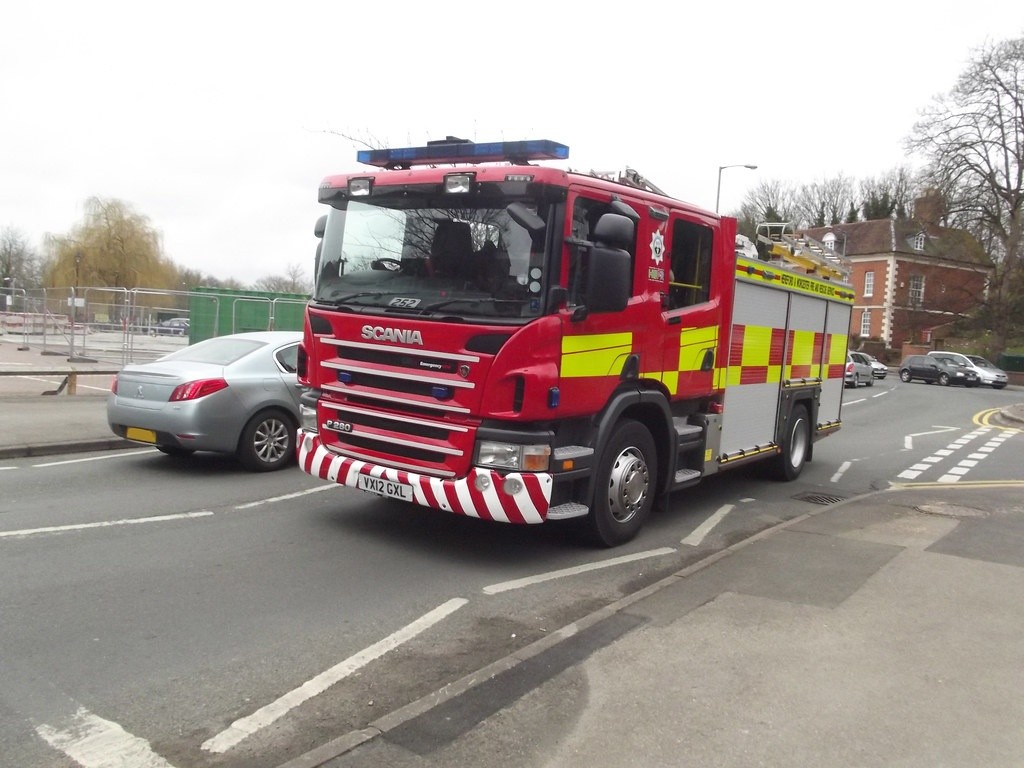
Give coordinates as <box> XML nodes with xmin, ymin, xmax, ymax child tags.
<box><xmin>926</xmin><ymin>351</ymin><xmax>1008</xmax><ymax>389</ymax></box>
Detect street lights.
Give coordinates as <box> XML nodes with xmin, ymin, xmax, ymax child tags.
<box><xmin>715</xmin><ymin>163</ymin><xmax>757</xmax><ymax>215</ymax></box>
<box><xmin>72</xmin><ymin>251</ymin><xmax>82</xmax><ymax>322</ymax></box>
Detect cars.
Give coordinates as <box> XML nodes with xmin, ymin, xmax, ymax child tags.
<box><xmin>864</xmin><ymin>354</ymin><xmax>888</xmax><ymax>380</ymax></box>
<box><xmin>149</xmin><ymin>317</ymin><xmax>190</xmax><ymax>336</ymax></box>
<box><xmin>845</xmin><ymin>353</ymin><xmax>875</xmax><ymax>387</ymax></box>
<box><xmin>898</xmin><ymin>354</ymin><xmax>981</xmax><ymax>387</ymax></box>
<box><xmin>106</xmin><ymin>329</ymin><xmax>307</xmax><ymax>473</ymax></box>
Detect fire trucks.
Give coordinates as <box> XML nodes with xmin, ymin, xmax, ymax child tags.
<box><xmin>295</xmin><ymin>136</ymin><xmax>856</xmax><ymax>549</ymax></box>
